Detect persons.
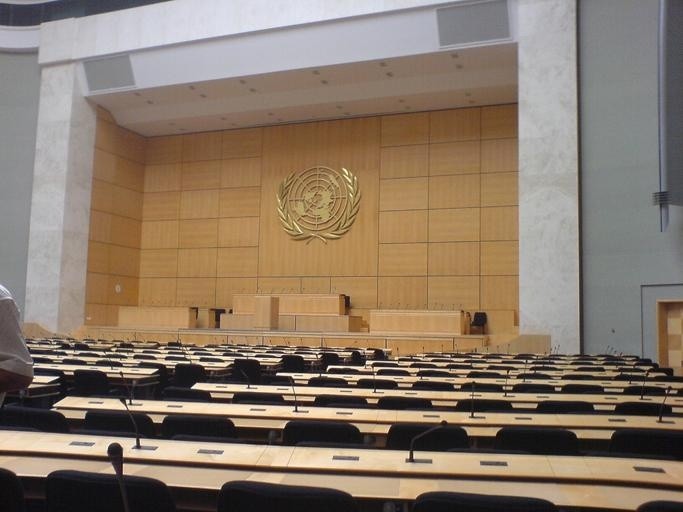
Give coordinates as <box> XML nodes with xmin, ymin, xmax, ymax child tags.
<box><xmin>0</xmin><ymin>284</ymin><xmax>35</xmax><ymax>395</ymax></box>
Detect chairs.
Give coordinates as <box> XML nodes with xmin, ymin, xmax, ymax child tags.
<box><xmin>471</xmin><ymin>311</ymin><xmax>488</xmax><ymax>334</ymax></box>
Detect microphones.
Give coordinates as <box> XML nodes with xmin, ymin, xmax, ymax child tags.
<box><xmin>656</xmin><ymin>385</ymin><xmax>675</xmax><ymax>424</ymax></box>
<box><xmin>534</xmin><ymin>355</ymin><xmax>540</xmax><ymax>374</ymax></box>
<box><xmin>177</xmin><ymin>334</ymin><xmax>327</xmax><ymax>358</ymax></box>
<box><xmin>288</xmin><ymin>376</ymin><xmax>309</xmax><ymax>413</ymax></box>
<box><xmin>469</xmin><ymin>381</ymin><xmax>485</xmax><ymax>418</ymax></box>
<box><xmin>638</xmin><ymin>372</ymin><xmax>654</xmax><ymax>400</ymax></box>
<box><xmin>503</xmin><ymin>367</ymin><xmax>516</xmax><ymax>397</ymax></box>
<box><xmin>543</xmin><ymin>345</ymin><xmax>560</xmax><ymax>367</ymax></box>
<box><xmin>103</xmin><ymin>350</ymin><xmax>118</xmax><ymax>370</ymax></box>
<box><xmin>628</xmin><ymin>362</ymin><xmax>638</xmax><ymax>385</ymax></box>
<box><xmin>316</xmin><ymin>354</ymin><xmax>326</xmax><ymax>377</ymax></box>
<box><xmin>106</xmin><ymin>439</ymin><xmax>132</xmax><ymax>511</ymax></box>
<box><xmin>119</xmin><ymin>372</ymin><xmax>141</xmax><ymax>406</ymax></box>
<box><xmin>27</xmin><ymin>330</ymin><xmax>147</xmax><ymax>361</ymax></box>
<box><xmin>371</xmin><ymin>365</ymin><xmax>383</xmax><ymax>393</ymax></box>
<box><xmin>522</xmin><ymin>360</ymin><xmax>532</xmax><ymax>382</ymax></box>
<box><xmin>120</xmin><ymin>398</ymin><xmax>158</xmax><ymax>450</ymax></box>
<box><xmin>232</xmin><ymin>360</ymin><xmax>258</xmax><ymax>389</ymax></box>
<box><xmin>363</xmin><ymin>351</ymin><xmax>371</xmax><ymax>369</ymax></box>
<box><xmin>184</xmin><ymin>345</ymin><xmax>192</xmax><ymax>364</ymax></box>
<box><xmin>405</xmin><ymin>420</ymin><xmax>448</xmax><ymax>463</ymax></box>
<box><xmin>397</xmin><ymin>343</ymin><xmax>511</xmax><ymax>380</ymax></box>
<box><xmin>604</xmin><ymin>344</ymin><xmax>628</xmax><ymax>374</ymax></box>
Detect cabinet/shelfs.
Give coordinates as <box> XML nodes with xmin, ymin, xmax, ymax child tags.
<box><xmin>656</xmin><ymin>298</ymin><xmax>683</xmax><ymax>380</ymax></box>
